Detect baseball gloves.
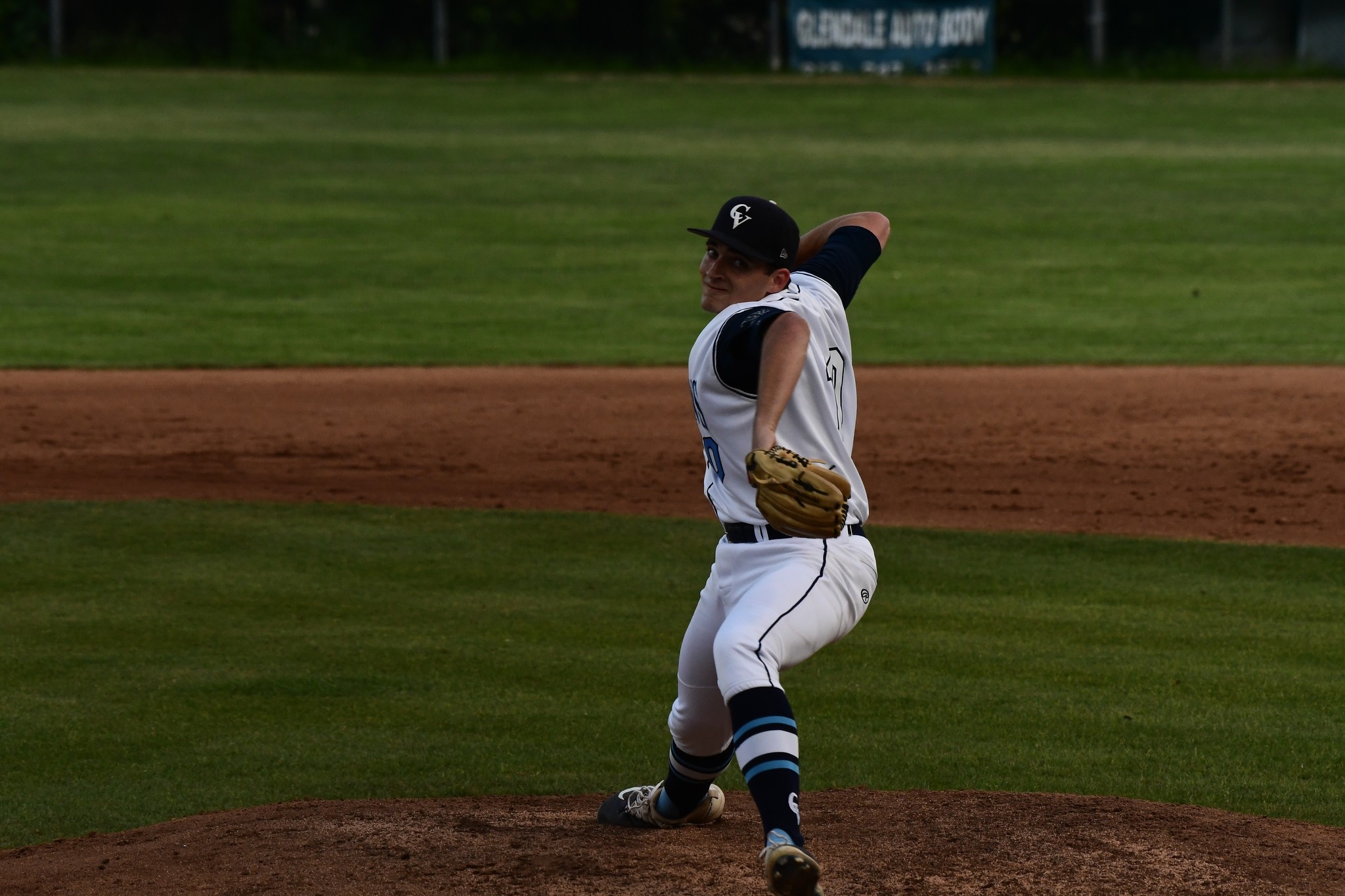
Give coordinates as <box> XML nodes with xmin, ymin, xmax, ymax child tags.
<box><xmin>746</xmin><ymin>446</ymin><xmax>851</xmax><ymax>540</ymax></box>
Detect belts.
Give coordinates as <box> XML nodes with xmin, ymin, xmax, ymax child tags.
<box><xmin>724</xmin><ymin>515</ymin><xmax>869</xmax><ymax>544</ymax></box>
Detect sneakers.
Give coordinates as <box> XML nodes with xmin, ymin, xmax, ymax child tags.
<box><xmin>596</xmin><ymin>783</ymin><xmax>726</xmax><ymax>826</ymax></box>
<box><xmin>759</xmin><ymin>831</ymin><xmax>822</xmax><ymax>896</ymax></box>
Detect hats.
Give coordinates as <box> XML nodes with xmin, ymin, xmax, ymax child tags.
<box><xmin>686</xmin><ymin>195</ymin><xmax>799</xmax><ymax>270</ymax></box>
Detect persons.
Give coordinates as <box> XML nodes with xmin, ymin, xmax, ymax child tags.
<box><xmin>597</xmin><ymin>198</ymin><xmax>892</xmax><ymax>896</ymax></box>
